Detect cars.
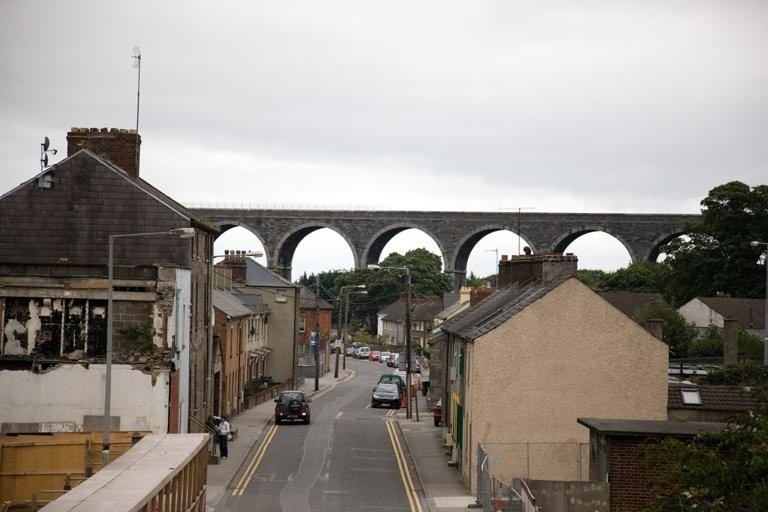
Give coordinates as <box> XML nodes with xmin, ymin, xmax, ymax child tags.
<box><xmin>421</xmin><ymin>366</ymin><xmax>430</xmax><ymax>396</ymax></box>
<box><xmin>431</xmin><ymin>396</ymin><xmax>442</xmax><ymax>428</ymax></box>
<box><xmin>328</xmin><ymin>338</ymin><xmax>421</xmax><ymax>409</ymax></box>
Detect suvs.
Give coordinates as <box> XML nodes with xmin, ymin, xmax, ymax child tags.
<box><xmin>273</xmin><ymin>389</ymin><xmax>312</xmax><ymax>425</ymax></box>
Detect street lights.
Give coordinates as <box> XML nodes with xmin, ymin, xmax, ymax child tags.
<box><xmin>333</xmin><ymin>283</ymin><xmax>369</xmax><ymax>378</ymax></box>
<box><xmin>102</xmin><ymin>227</ymin><xmax>196</xmax><ymax>467</ymax></box>
<box><xmin>366</xmin><ymin>264</ymin><xmax>415</xmax><ymax>420</ymax></box>
<box><xmin>205</xmin><ymin>249</ymin><xmax>268</xmax><ymax>414</ymax></box>
<box><xmin>342</xmin><ymin>290</ymin><xmax>368</xmax><ymax>371</ymax></box>
<box><xmin>273</xmin><ymin>285</ymin><xmax>298</xmax><ymax>391</ymax></box>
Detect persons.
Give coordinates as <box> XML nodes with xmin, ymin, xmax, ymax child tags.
<box><xmin>216</xmin><ymin>415</ymin><xmax>230</xmax><ymax>459</ymax></box>
<box><xmin>204</xmin><ymin>414</ymin><xmax>221</xmax><ymax>452</ymax></box>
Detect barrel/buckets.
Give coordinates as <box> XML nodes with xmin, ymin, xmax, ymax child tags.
<box><xmin>432</xmin><ymin>405</ymin><xmax>442</xmax><ymax>427</ymax></box>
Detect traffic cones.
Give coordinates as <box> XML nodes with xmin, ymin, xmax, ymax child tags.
<box><xmin>492</xmin><ymin>480</ymin><xmax>504</xmax><ymax>510</ymax></box>
